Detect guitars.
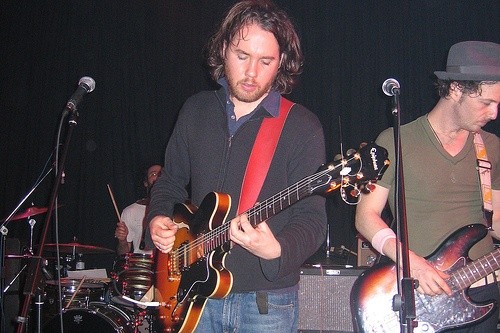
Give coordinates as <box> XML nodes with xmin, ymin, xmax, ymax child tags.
<box><xmin>145</xmin><ymin>139</ymin><xmax>391</xmax><ymax>333</ymax></box>
<box><xmin>348</xmin><ymin>222</ymin><xmax>500</xmax><ymax>333</ymax></box>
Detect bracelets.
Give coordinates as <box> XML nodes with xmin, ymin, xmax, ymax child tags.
<box><xmin>371</xmin><ymin>228</ymin><xmax>396</xmax><ymax>256</ymax></box>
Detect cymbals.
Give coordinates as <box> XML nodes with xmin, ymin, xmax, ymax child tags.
<box><xmin>40</xmin><ymin>241</ymin><xmax>119</xmax><ymax>254</ymax></box>
<box><xmin>0</xmin><ymin>204</ymin><xmax>67</xmax><ymax>222</ymax></box>
<box><xmin>1</xmin><ymin>253</ymin><xmax>48</xmax><ymax>260</ymax></box>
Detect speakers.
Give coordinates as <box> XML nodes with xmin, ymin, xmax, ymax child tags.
<box><xmin>1</xmin><ymin>237</ymin><xmax>23</xmax><ymax>333</ymax></box>
<box><xmin>296</xmin><ymin>267</ymin><xmax>368</xmax><ymax>333</ymax></box>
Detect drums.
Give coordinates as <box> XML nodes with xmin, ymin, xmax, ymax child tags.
<box><xmin>109</xmin><ymin>252</ymin><xmax>157</xmax><ymax>308</ymax></box>
<box><xmin>43</xmin><ymin>300</ymin><xmax>139</xmax><ymax>333</ymax></box>
<box><xmin>43</xmin><ymin>277</ymin><xmax>108</xmax><ymax>308</ymax></box>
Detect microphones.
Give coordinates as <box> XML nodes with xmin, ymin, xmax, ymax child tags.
<box><xmin>382</xmin><ymin>78</ymin><xmax>401</xmax><ymax>97</ymax></box>
<box><xmin>62</xmin><ymin>76</ymin><xmax>96</xmax><ymax>116</ymax></box>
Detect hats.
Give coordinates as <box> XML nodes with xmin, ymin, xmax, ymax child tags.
<box><xmin>433</xmin><ymin>40</ymin><xmax>500</xmax><ymax>81</ymax></box>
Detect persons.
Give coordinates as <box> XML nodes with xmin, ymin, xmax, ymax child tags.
<box><xmin>146</xmin><ymin>0</ymin><xmax>328</xmax><ymax>333</ymax></box>
<box><xmin>114</xmin><ymin>164</ymin><xmax>161</xmax><ymax>258</ymax></box>
<box><xmin>355</xmin><ymin>42</ymin><xmax>500</xmax><ymax>333</ymax></box>
